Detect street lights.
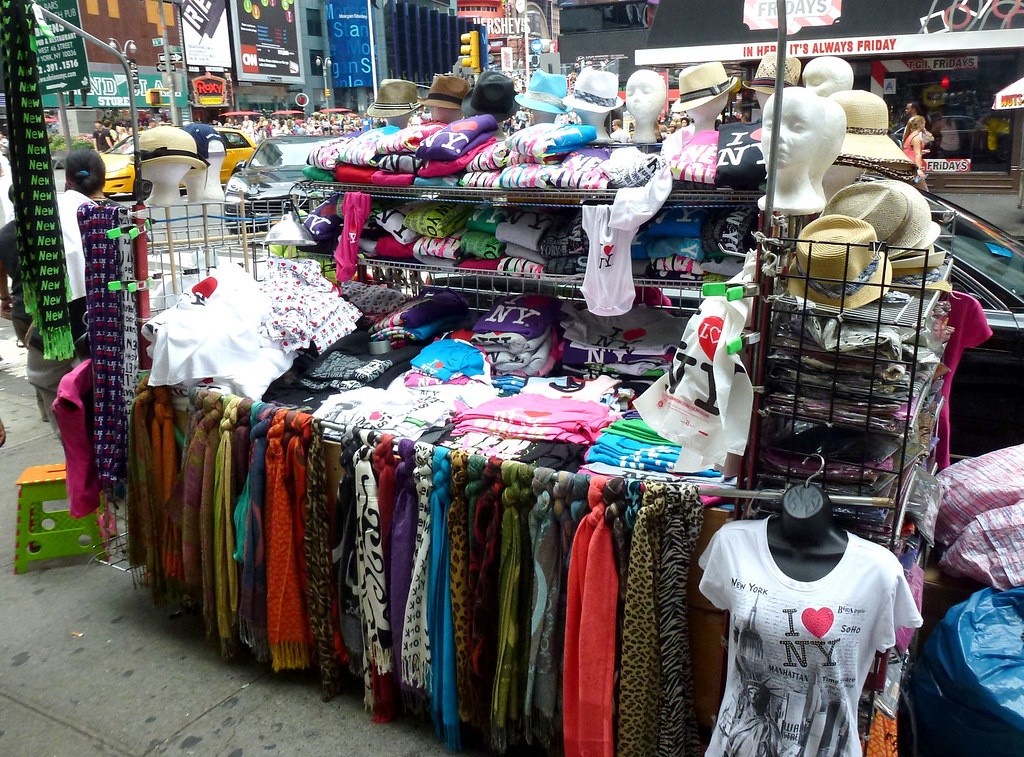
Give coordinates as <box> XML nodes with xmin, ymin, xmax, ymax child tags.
<box><xmin>315</xmin><ymin>55</ymin><xmax>333</xmax><ymax>133</ymax></box>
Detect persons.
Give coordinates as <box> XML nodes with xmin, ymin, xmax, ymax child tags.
<box><xmin>561</xmin><ymin>87</ymin><xmax>577</xmax><ymax>125</ymax></box>
<box><xmin>210</xmin><ymin>115</ymin><xmax>387</xmax><ymax>140</ymax></box>
<box><xmin>367</xmin><ymin>79</ymin><xmax>423</xmax><ymax>128</ymax></box>
<box><xmin>92</xmin><ymin>117</ymin><xmax>173</xmax><ymax>153</ymax></box>
<box><xmin>129</xmin><ymin>124</ymin><xmax>226</xmax><ymax>218</ymax></box>
<box><xmin>698</xmin><ymin>484</ymin><xmax>924</xmax><ymax>757</ymax></box>
<box><xmin>902</xmin><ymin>103</ymin><xmax>929</xmax><ymax>190</ymax></box>
<box><xmin>742</xmin><ymin>52</ymin><xmax>918</xmax><ymax>214</ymax></box>
<box><xmin>563</xmin><ymin>68</ymin><xmax>623</xmax><ymax>141</ymax></box>
<box><xmin>409</xmin><ymin>111</ymin><xmax>428</xmax><ymax>126</ymax></box>
<box><xmin>604</xmin><ymin>119</ymin><xmax>634</xmax><ymax>143</ymax></box>
<box><xmin>515</xmin><ymin>69</ymin><xmax>574</xmax><ymax>123</ymax></box>
<box><xmin>672</xmin><ymin>61</ymin><xmax>738</xmax><ymax>130</ymax></box>
<box><xmin>655</xmin><ymin>110</ymin><xmax>694</xmax><ymax>143</ymax></box>
<box><xmin>502</xmin><ymin>70</ymin><xmax>529</xmax><ymax>135</ymax></box>
<box><xmin>625</xmin><ymin>69</ymin><xmax>666</xmax><ymax>143</ymax></box>
<box><xmin>418</xmin><ymin>72</ymin><xmax>520</xmax><ymax>138</ymax></box>
<box><xmin>0</xmin><ymin>133</ymin><xmax>125</xmax><ymax>438</ymax></box>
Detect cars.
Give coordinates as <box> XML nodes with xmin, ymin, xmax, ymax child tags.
<box><xmin>893</xmin><ymin>114</ymin><xmax>1014</xmax><ymax>166</ymax></box>
<box><xmin>860</xmin><ymin>170</ymin><xmax>1024</xmax><ymax>466</ymax></box>
<box><xmin>222</xmin><ymin>135</ymin><xmax>352</xmax><ymax>238</ymax></box>
<box><xmin>91</xmin><ymin>125</ymin><xmax>259</xmax><ymax>202</ymax></box>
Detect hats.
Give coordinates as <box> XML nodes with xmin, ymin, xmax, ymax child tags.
<box><xmin>828</xmin><ymin>90</ymin><xmax>918</xmax><ymax>180</ymax></box>
<box><xmin>367</xmin><ymin>78</ymin><xmax>423</xmax><ymax>118</ymax></box>
<box><xmin>788</xmin><ymin>215</ymin><xmax>893</xmax><ymax>310</ymax></box>
<box><xmin>461</xmin><ymin>71</ymin><xmax>520</xmax><ymax>123</ymax></box>
<box><xmin>672</xmin><ymin>62</ymin><xmax>738</xmax><ymax>113</ymax></box>
<box><xmin>819</xmin><ymin>180</ymin><xmax>946</xmax><ymax>276</ymax></box>
<box><xmin>181</xmin><ymin>123</ymin><xmax>226</xmax><ymax>159</ymax></box>
<box><xmin>514</xmin><ymin>69</ymin><xmax>567</xmax><ymax>114</ymax></box>
<box><xmin>138</xmin><ymin>126</ymin><xmax>210</xmax><ymax>176</ymax></box>
<box><xmin>743</xmin><ymin>52</ymin><xmax>802</xmax><ymax>96</ymax></box>
<box><xmin>417</xmin><ymin>76</ymin><xmax>470</xmax><ymax>109</ymax></box>
<box><xmin>562</xmin><ymin>68</ymin><xmax>624</xmax><ymax>114</ymax></box>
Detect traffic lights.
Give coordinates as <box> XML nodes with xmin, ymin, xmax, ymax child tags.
<box><xmin>459</xmin><ymin>31</ymin><xmax>478</xmax><ymax>71</ymax></box>
<box><xmin>149</xmin><ymin>90</ymin><xmax>161</xmax><ymax>106</ymax></box>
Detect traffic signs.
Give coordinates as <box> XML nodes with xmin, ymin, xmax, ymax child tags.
<box><xmin>155</xmin><ymin>62</ymin><xmax>177</xmax><ymax>73</ymax></box>
<box><xmin>28</xmin><ymin>0</ymin><xmax>93</xmax><ymax>94</ymax></box>
<box><xmin>158</xmin><ymin>52</ymin><xmax>184</xmax><ymax>63</ymax></box>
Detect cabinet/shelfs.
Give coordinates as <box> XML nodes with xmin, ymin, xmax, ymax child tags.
<box><xmin>306</xmin><ymin>179</ymin><xmax>764</xmax><ymax>315</ymax></box>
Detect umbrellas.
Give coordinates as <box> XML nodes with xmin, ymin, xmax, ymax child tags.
<box><xmin>992</xmin><ymin>77</ymin><xmax>1024</xmax><ymax>109</ymax></box>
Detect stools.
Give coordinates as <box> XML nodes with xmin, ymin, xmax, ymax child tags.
<box><xmin>15</xmin><ymin>463</ymin><xmax>116</xmax><ymax>576</ymax></box>
